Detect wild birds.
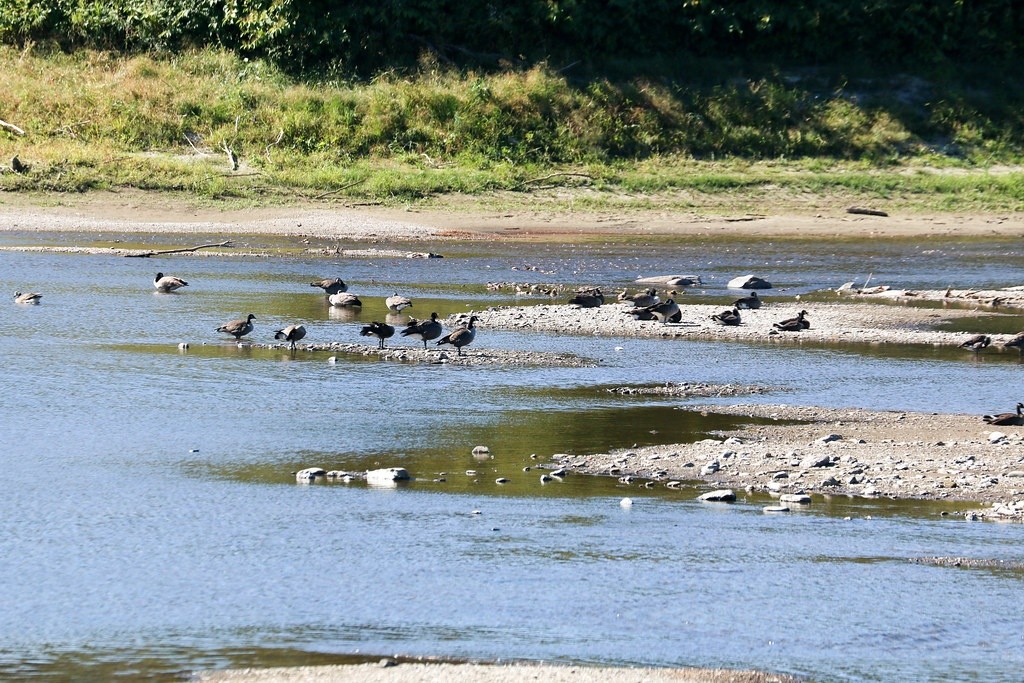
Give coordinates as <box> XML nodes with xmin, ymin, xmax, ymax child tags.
<box><xmin>14</xmin><ymin>271</ymin><xmax>1022</xmax><ymax>425</ymax></box>
